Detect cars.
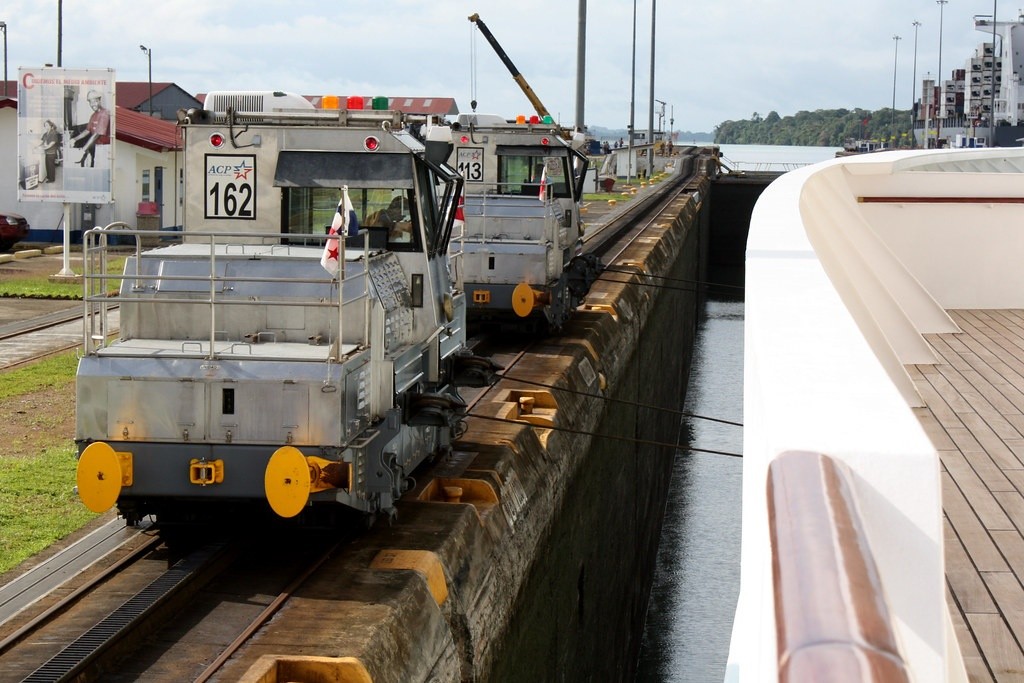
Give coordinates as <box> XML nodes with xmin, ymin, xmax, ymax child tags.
<box><xmin>0</xmin><ymin>211</ymin><xmax>31</xmax><ymax>250</ymax></box>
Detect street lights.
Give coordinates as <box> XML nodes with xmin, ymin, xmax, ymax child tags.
<box><xmin>911</xmin><ymin>20</ymin><xmax>922</xmax><ymax>148</ymax></box>
<box><xmin>891</xmin><ymin>35</ymin><xmax>903</xmax><ymax>125</ymax></box>
<box><xmin>935</xmin><ymin>0</ymin><xmax>949</xmax><ymax>148</ymax></box>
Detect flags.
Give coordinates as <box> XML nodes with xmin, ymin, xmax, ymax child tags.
<box><xmin>321</xmin><ymin>188</ymin><xmax>358</xmax><ymax>278</ymax></box>
<box><xmin>445</xmin><ymin>171</ymin><xmax>465</xmax><ymax>228</ymax></box>
<box><xmin>539</xmin><ymin>165</ymin><xmax>546</xmax><ymax>203</ymax></box>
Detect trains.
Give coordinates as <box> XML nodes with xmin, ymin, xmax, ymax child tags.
<box><xmin>412</xmin><ymin>110</ymin><xmax>604</xmax><ymax>336</ymax></box>
<box><xmin>71</xmin><ymin>88</ymin><xmax>504</xmax><ymax>552</ymax></box>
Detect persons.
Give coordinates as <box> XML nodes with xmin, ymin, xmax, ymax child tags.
<box><xmin>524</xmin><ymin>163</ymin><xmax>550</xmax><ymax>183</ymax></box>
<box><xmin>667</xmin><ymin>141</ymin><xmax>674</xmax><ymax>156</ymax></box>
<box><xmin>619</xmin><ymin>138</ymin><xmax>623</xmax><ymax>147</ymax></box>
<box><xmin>602</xmin><ymin>141</ymin><xmax>610</xmax><ymax>154</ymax></box>
<box><xmin>34</xmin><ymin>121</ymin><xmax>58</xmax><ymax>183</ymax></box>
<box><xmin>614</xmin><ymin>142</ymin><xmax>618</xmax><ymax>148</ymax></box>
<box><xmin>362</xmin><ymin>196</ymin><xmax>411</xmax><ymax>238</ymax></box>
<box><xmin>68</xmin><ymin>90</ymin><xmax>109</xmax><ymax>162</ymax></box>
